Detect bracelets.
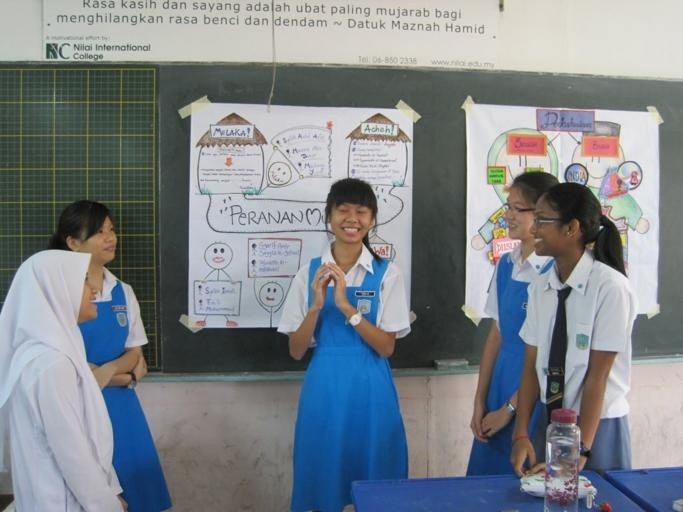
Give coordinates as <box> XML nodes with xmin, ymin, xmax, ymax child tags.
<box><xmin>580</xmin><ymin>442</ymin><xmax>592</xmax><ymax>458</ymax></box>
<box><xmin>511</xmin><ymin>435</ymin><xmax>528</xmax><ymax>446</ymax></box>
<box><xmin>505</xmin><ymin>400</ymin><xmax>516</xmax><ymax>417</ymax></box>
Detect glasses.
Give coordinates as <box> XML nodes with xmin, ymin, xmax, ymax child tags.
<box><xmin>503</xmin><ymin>203</ymin><xmax>536</xmax><ymax>214</ymax></box>
<box><xmin>533</xmin><ymin>216</ymin><xmax>565</xmax><ymax>227</ymax></box>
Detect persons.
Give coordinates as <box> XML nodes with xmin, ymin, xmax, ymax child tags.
<box><xmin>49</xmin><ymin>200</ymin><xmax>173</xmax><ymax>511</ymax></box>
<box><xmin>509</xmin><ymin>182</ymin><xmax>638</xmax><ymax>478</ymax></box>
<box><xmin>465</xmin><ymin>170</ymin><xmax>559</xmax><ymax>477</ymax></box>
<box><xmin>0</xmin><ymin>249</ymin><xmax>128</xmax><ymax>512</ymax></box>
<box><xmin>277</xmin><ymin>178</ymin><xmax>408</xmax><ymax>512</ymax></box>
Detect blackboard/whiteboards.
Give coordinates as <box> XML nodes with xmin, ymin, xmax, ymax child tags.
<box><xmin>0</xmin><ymin>61</ymin><xmax>683</xmax><ymax>383</ymax></box>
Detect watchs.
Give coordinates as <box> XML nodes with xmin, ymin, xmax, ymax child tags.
<box><xmin>126</xmin><ymin>371</ymin><xmax>136</xmax><ymax>390</ymax></box>
<box><xmin>344</xmin><ymin>311</ymin><xmax>362</xmax><ymax>327</ymax></box>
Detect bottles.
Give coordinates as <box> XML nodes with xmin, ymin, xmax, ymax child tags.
<box><xmin>544</xmin><ymin>409</ymin><xmax>580</xmax><ymax>512</ymax></box>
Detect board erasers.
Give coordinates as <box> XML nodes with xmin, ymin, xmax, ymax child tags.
<box><xmin>434</xmin><ymin>358</ymin><xmax>469</xmax><ymax>370</ymax></box>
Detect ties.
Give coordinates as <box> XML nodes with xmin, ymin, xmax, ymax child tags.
<box><xmin>547</xmin><ymin>287</ymin><xmax>572</xmax><ymax>421</ymax></box>
